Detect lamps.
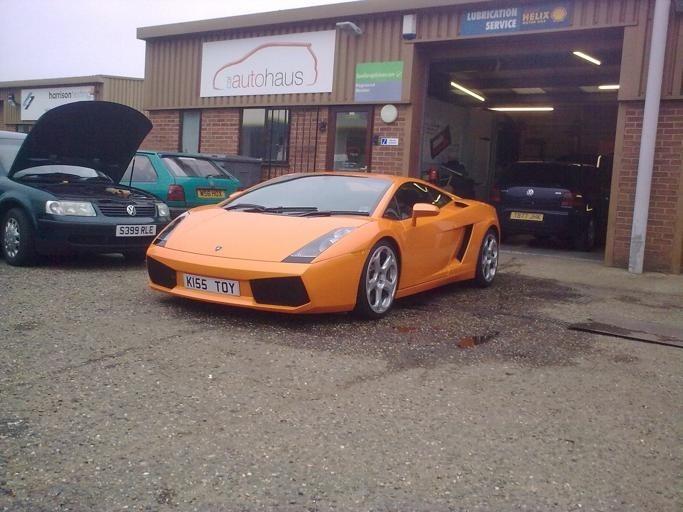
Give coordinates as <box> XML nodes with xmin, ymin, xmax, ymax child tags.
<box><xmin>337</xmin><ymin>21</ymin><xmax>362</xmax><ymax>37</ymax></box>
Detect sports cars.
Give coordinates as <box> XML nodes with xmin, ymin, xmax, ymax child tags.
<box><xmin>145</xmin><ymin>171</ymin><xmax>501</xmax><ymax>321</ymax></box>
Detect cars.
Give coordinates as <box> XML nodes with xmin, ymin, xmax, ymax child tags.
<box><xmin>484</xmin><ymin>153</ymin><xmax>614</xmax><ymax>251</ymax></box>
<box><xmin>0</xmin><ymin>101</ymin><xmax>172</xmax><ymax>267</ymax></box>
<box><xmin>118</xmin><ymin>150</ymin><xmax>241</xmax><ymax>222</ymax></box>
